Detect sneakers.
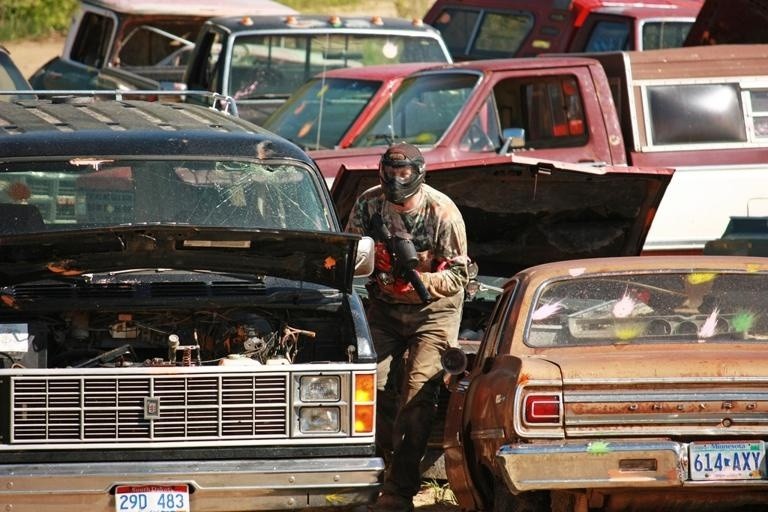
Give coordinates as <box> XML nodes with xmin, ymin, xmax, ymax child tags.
<box><xmin>367</xmin><ymin>494</ymin><xmax>414</xmax><ymax>512</ymax></box>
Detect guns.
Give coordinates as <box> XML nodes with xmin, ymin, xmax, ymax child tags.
<box><xmin>370</xmin><ymin>212</ymin><xmax>432</xmax><ymax>305</ymax></box>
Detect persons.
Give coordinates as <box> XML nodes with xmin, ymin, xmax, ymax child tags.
<box><xmin>343</xmin><ymin>141</ymin><xmax>470</xmax><ymax>511</ymax></box>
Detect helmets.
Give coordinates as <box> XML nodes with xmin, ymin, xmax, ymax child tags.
<box><xmin>378</xmin><ymin>144</ymin><xmax>426</xmax><ymax>204</ymax></box>
